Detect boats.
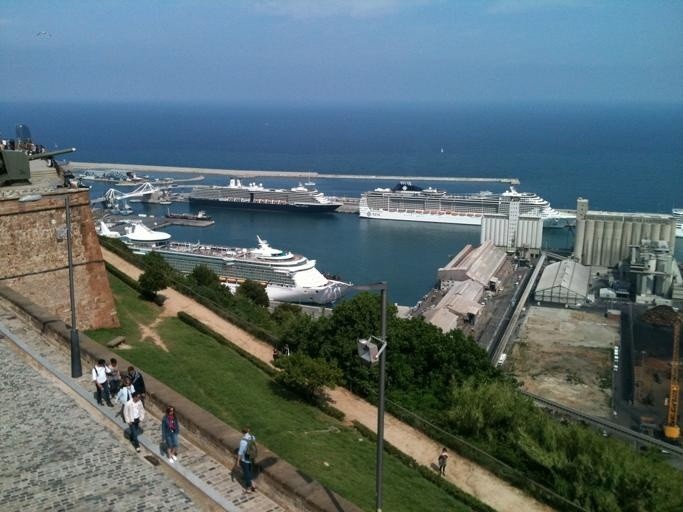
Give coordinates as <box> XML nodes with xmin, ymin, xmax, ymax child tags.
<box><xmin>70</xmin><ymin>166</ymin><xmax>175</xmax><ymax>215</ymax></box>
<box><xmin>95</xmin><ymin>217</ymin><xmax>355</xmax><ymax>317</ymax></box>
<box><xmin>160</xmin><ymin>207</ymin><xmax>215</xmax><ymax>228</ymax></box>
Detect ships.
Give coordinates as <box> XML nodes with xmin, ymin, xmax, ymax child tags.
<box><xmin>356</xmin><ymin>177</ymin><xmax>567</xmax><ymax>228</ymax></box>
<box><xmin>187</xmin><ymin>167</ymin><xmax>344</xmax><ymax>213</ymax></box>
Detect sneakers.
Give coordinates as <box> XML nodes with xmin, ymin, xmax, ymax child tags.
<box><xmin>136</xmin><ymin>448</ymin><xmax>140</xmax><ymax>452</ymax></box>
<box><xmin>169</xmin><ymin>455</ymin><xmax>178</xmax><ymax>464</ymax></box>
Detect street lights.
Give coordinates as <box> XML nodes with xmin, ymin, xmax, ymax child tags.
<box><xmin>51</xmin><ymin>192</ymin><xmax>84</xmax><ymax>376</ymax></box>
<box><xmin>356</xmin><ymin>280</ymin><xmax>387</xmax><ymax>512</ymax></box>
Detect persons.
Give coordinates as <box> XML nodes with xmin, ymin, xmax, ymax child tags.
<box><xmin>90</xmin><ymin>358</ymin><xmax>113</xmax><ymax>407</ymax></box>
<box><xmin>161</xmin><ymin>405</ymin><xmax>181</xmax><ymax>463</ymax></box>
<box><xmin>438</xmin><ymin>447</ymin><xmax>448</xmax><ymax>477</ymax></box>
<box><xmin>127</xmin><ymin>366</ymin><xmax>145</xmax><ymax>408</ymax></box>
<box><xmin>271</xmin><ymin>347</ymin><xmax>279</xmax><ymax>360</ymax></box>
<box><xmin>107</xmin><ymin>357</ymin><xmax>122</xmax><ymax>398</ymax></box>
<box><xmin>113</xmin><ymin>376</ymin><xmax>136</xmax><ymax>424</ymax></box>
<box><xmin>122</xmin><ymin>391</ymin><xmax>145</xmax><ymax>452</ymax></box>
<box><xmin>236</xmin><ymin>425</ymin><xmax>260</xmax><ymax>494</ymax></box>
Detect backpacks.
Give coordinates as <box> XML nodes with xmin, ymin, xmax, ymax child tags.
<box><xmin>240</xmin><ymin>436</ymin><xmax>257</xmax><ymax>461</ymax></box>
